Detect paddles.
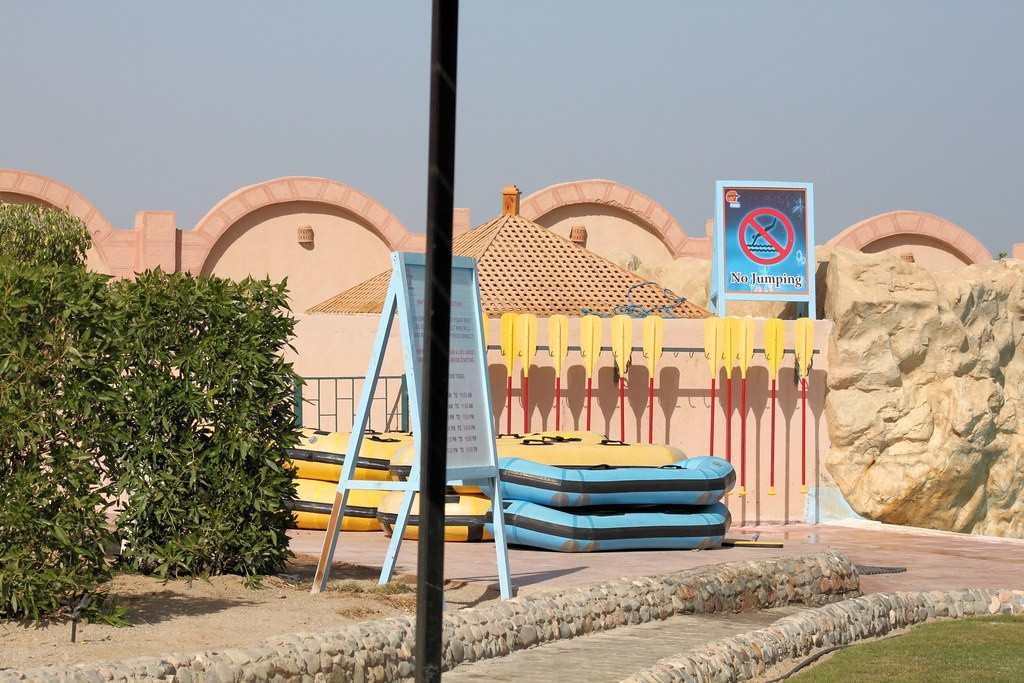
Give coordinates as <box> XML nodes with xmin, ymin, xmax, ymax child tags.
<box><xmin>578</xmin><ymin>314</ymin><xmax>603</xmax><ymax>432</ymax></box>
<box><xmin>610</xmin><ymin>314</ymin><xmax>633</xmax><ymax>444</ymax></box>
<box><xmin>481</xmin><ymin>312</ymin><xmax>539</xmax><ymax>435</ymax></box>
<box><xmin>793</xmin><ymin>317</ymin><xmax>815</xmax><ymax>494</ymax></box>
<box><xmin>547</xmin><ymin>314</ymin><xmax>569</xmax><ymax>432</ymax></box>
<box><xmin>702</xmin><ymin>317</ymin><xmax>756</xmax><ymax>498</ymax></box>
<box><xmin>641</xmin><ymin>316</ymin><xmax>663</xmax><ymax>444</ymax></box>
<box><xmin>763</xmin><ymin>318</ymin><xmax>785</xmax><ymax>495</ymax></box>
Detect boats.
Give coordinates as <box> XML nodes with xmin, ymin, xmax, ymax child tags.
<box><xmin>271</xmin><ymin>425</ymin><xmax>736</xmax><ymax>554</ymax></box>
